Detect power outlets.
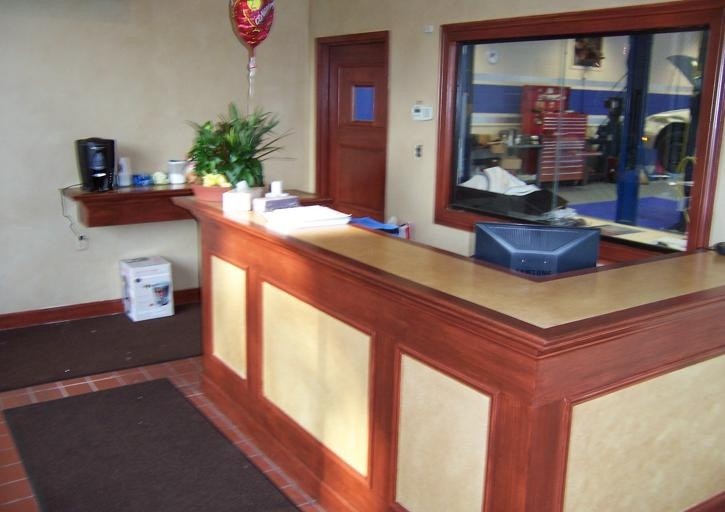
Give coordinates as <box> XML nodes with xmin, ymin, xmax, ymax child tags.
<box><xmin>74</xmin><ymin>235</ymin><xmax>90</xmax><ymax>252</ymax></box>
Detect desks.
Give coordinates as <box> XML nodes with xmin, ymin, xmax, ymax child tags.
<box><xmin>172</xmin><ymin>195</ymin><xmax>725</xmax><ymax>512</ymax></box>
<box><xmin>59</xmin><ymin>182</ymin><xmax>196</xmax><ymax>228</ymax></box>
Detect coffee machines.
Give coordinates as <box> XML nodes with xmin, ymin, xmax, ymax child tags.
<box><xmin>76</xmin><ymin>137</ymin><xmax>115</xmax><ymax>193</ymax></box>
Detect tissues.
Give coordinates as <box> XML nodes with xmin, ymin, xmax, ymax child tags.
<box><xmin>221</xmin><ymin>181</ymin><xmax>269</xmax><ymax>212</ymax></box>
<box><xmin>252</xmin><ymin>180</ymin><xmax>301</xmax><ymax>212</ymax></box>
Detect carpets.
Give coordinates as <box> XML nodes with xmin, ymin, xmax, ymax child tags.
<box><xmin>3</xmin><ymin>378</ymin><xmax>301</xmax><ymax>512</ymax></box>
<box><xmin>0</xmin><ymin>300</ymin><xmax>201</xmax><ymax>390</ymax></box>
<box><xmin>567</xmin><ymin>196</ymin><xmax>684</xmax><ymax>230</ymax></box>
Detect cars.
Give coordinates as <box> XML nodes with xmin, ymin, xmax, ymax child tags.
<box><xmin>643</xmin><ymin>107</ymin><xmax>693</xmax><ymax>174</ymax></box>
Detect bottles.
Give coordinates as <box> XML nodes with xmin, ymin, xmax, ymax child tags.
<box><xmin>116</xmin><ymin>157</ymin><xmax>132</xmax><ymax>187</ymax></box>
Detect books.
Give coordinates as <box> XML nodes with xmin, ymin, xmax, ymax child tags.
<box><xmin>265</xmin><ymin>205</ymin><xmax>352</xmax><ymax>235</ymax></box>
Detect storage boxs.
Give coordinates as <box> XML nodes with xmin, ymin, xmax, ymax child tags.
<box><xmin>121</xmin><ymin>256</ymin><xmax>176</xmax><ymax>323</ymax></box>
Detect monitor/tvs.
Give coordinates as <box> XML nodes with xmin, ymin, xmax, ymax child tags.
<box><xmin>476</xmin><ymin>222</ymin><xmax>600</xmax><ymax>276</ymax></box>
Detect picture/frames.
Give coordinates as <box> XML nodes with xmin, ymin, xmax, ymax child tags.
<box><xmin>570</xmin><ymin>38</ymin><xmax>602</xmax><ymax>71</ymax></box>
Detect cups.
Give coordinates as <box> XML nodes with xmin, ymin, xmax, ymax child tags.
<box><xmin>167</xmin><ymin>161</ymin><xmax>186</xmax><ymax>184</ymax></box>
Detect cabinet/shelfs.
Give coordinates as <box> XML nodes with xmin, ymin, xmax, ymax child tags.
<box><xmin>522</xmin><ymin>85</ymin><xmax>570</xmax><ymax>174</ymax></box>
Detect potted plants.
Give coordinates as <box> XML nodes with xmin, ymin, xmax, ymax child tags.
<box><xmin>181</xmin><ymin>102</ymin><xmax>295</xmax><ymax>202</ymax></box>
<box><xmin>235</xmin><ymin>155</ymin><xmax>237</xmax><ymax>159</ymax></box>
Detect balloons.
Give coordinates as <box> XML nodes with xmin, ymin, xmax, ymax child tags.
<box><xmin>229</xmin><ymin>0</ymin><xmax>275</xmax><ymax>49</ymax></box>
